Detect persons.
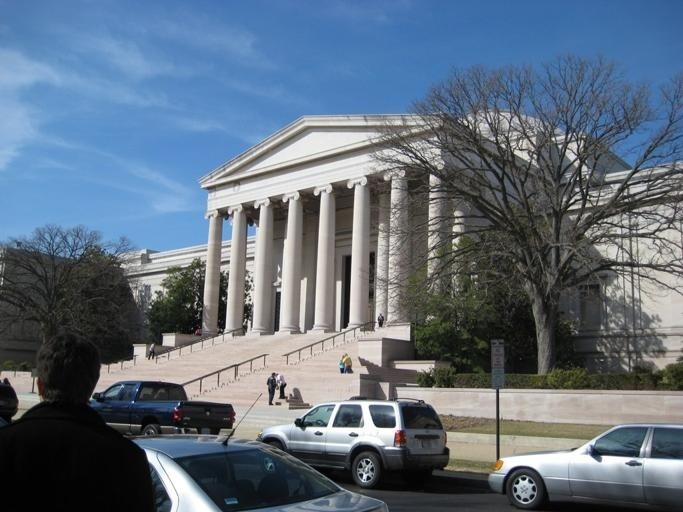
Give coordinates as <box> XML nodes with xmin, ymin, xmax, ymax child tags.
<box><xmin>267</xmin><ymin>372</ymin><xmax>277</xmax><ymax>405</ymax></box>
<box><xmin>194</xmin><ymin>324</ymin><xmax>224</xmax><ymax>336</ymax></box>
<box><xmin>0</xmin><ymin>332</ymin><xmax>157</xmax><ymax>512</ymax></box>
<box><xmin>275</xmin><ymin>374</ymin><xmax>287</xmax><ymax>399</ymax></box>
<box><xmin>378</xmin><ymin>314</ymin><xmax>384</xmax><ymax>327</ymax></box>
<box><xmin>0</xmin><ymin>379</ymin><xmax>19</xmax><ymax>426</ymax></box>
<box><xmin>343</xmin><ymin>353</ymin><xmax>352</xmax><ymax>373</ymax></box>
<box><xmin>338</xmin><ymin>354</ymin><xmax>344</xmax><ymax>373</ymax></box>
<box><xmin>148</xmin><ymin>342</ymin><xmax>155</xmax><ymax>360</ymax></box>
<box><xmin>242</xmin><ymin>314</ymin><xmax>253</xmax><ymax>334</ymax></box>
<box><xmin>2</xmin><ymin>377</ymin><xmax>11</xmax><ymax>385</ymax></box>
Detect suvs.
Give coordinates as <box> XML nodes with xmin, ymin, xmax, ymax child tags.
<box><xmin>254</xmin><ymin>396</ymin><xmax>450</xmax><ymax>489</ymax></box>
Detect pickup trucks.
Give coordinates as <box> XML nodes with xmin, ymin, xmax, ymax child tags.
<box><xmin>87</xmin><ymin>381</ymin><xmax>236</xmax><ymax>439</ymax></box>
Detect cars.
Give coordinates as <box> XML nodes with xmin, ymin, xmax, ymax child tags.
<box><xmin>119</xmin><ymin>392</ymin><xmax>391</xmax><ymax>512</ymax></box>
<box><xmin>487</xmin><ymin>424</ymin><xmax>682</xmax><ymax>512</ymax></box>
